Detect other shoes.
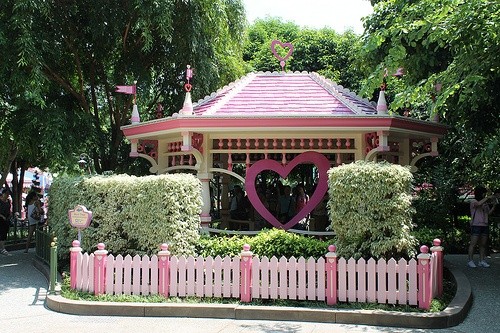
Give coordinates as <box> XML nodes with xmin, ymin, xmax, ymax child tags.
<box><xmin>24</xmin><ymin>250</ymin><xmax>29</xmax><ymax>253</ymax></box>
<box><xmin>469</xmin><ymin>261</ymin><xmax>477</xmax><ymax>267</ymax></box>
<box><xmin>479</xmin><ymin>261</ymin><xmax>490</xmax><ymax>268</ymax></box>
<box><xmin>1</xmin><ymin>249</ymin><xmax>8</xmax><ymax>255</ymax></box>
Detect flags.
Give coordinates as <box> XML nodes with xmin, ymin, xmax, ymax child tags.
<box><xmin>115</xmin><ymin>86</ymin><xmax>135</xmax><ymax>94</ymax></box>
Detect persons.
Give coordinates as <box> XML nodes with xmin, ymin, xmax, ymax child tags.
<box><xmin>256</xmin><ymin>180</ymin><xmax>310</xmax><ymax>231</ymax></box>
<box><xmin>311</xmin><ymin>178</ymin><xmax>330</xmax><ymax>232</ymax></box>
<box><xmin>24</xmin><ymin>191</ymin><xmax>42</xmax><ymax>253</ymax></box>
<box><xmin>466</xmin><ymin>186</ymin><xmax>496</xmax><ymax>267</ymax></box>
<box><xmin>0</xmin><ymin>189</ymin><xmax>13</xmax><ymax>255</ymax></box>
<box><xmin>231</xmin><ymin>185</ymin><xmax>251</xmax><ymax>231</ymax></box>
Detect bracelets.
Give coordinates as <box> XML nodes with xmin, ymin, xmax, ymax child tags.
<box><xmin>486</xmin><ymin>197</ymin><xmax>489</xmax><ymax>200</ymax></box>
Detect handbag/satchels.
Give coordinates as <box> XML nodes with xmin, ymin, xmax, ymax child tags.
<box><xmin>30</xmin><ymin>207</ymin><xmax>41</xmax><ymax>221</ymax></box>
<box><xmin>278</xmin><ymin>213</ymin><xmax>289</xmax><ymax>224</ymax></box>
